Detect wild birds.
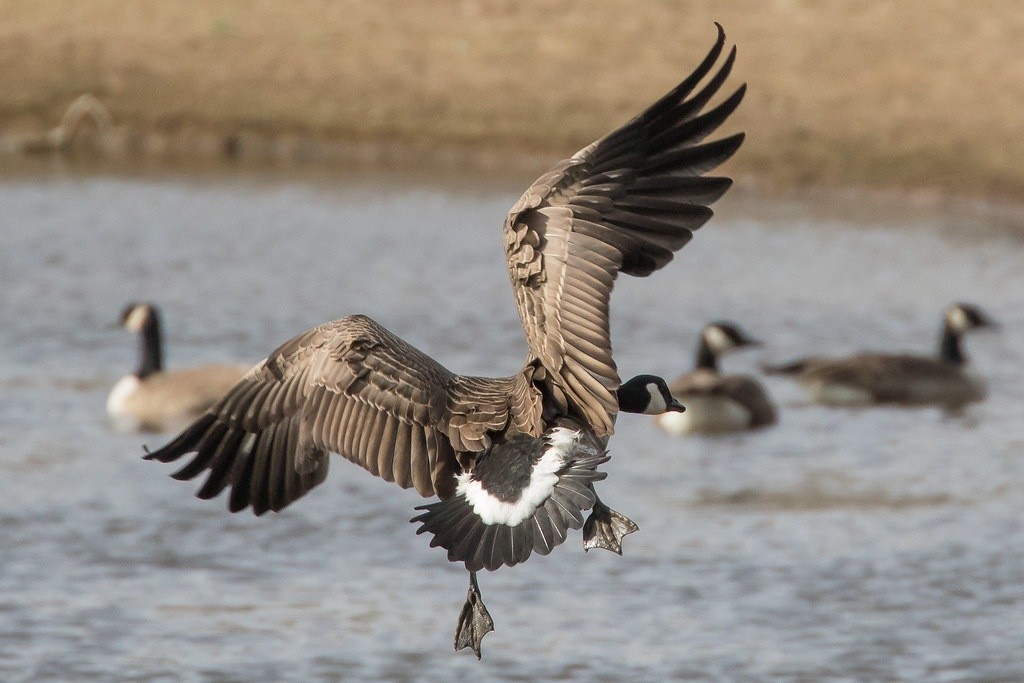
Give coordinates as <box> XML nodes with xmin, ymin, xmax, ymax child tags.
<box><xmin>102</xmin><ymin>21</ymin><xmax>1008</xmax><ymax>662</ymax></box>
